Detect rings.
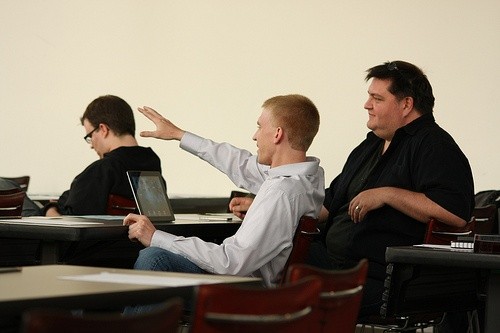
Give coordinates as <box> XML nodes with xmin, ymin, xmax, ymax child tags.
<box><xmin>356</xmin><ymin>206</ymin><xmax>360</xmax><ymax>212</ymax></box>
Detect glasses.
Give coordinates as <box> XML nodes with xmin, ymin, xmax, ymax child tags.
<box><xmin>389</xmin><ymin>63</ymin><xmax>411</xmax><ymax>84</ymax></box>
<box><xmin>84</xmin><ymin>122</ymin><xmax>110</xmax><ymax>144</ymax></box>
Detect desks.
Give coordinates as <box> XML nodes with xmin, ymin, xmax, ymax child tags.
<box><xmin>0</xmin><ymin>213</ymin><xmax>243</xmax><ymax>265</ymax></box>
<box><xmin>0</xmin><ymin>265</ymin><xmax>263</xmax><ymax>333</ymax></box>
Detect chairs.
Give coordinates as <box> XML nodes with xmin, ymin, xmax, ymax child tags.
<box><xmin>0</xmin><ymin>175</ymin><xmax>500</xmax><ymax>333</ymax></box>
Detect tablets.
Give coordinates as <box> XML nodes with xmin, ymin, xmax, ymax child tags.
<box><xmin>126</xmin><ymin>170</ymin><xmax>175</xmax><ymax>223</ymax></box>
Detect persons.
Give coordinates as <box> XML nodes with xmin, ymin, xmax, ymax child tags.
<box><xmin>0</xmin><ymin>95</ymin><xmax>166</xmax><ymax>216</ymax></box>
<box><xmin>229</xmin><ymin>60</ymin><xmax>474</xmax><ymax>323</ymax></box>
<box><xmin>121</xmin><ymin>94</ymin><xmax>326</xmax><ymax>318</ymax></box>
